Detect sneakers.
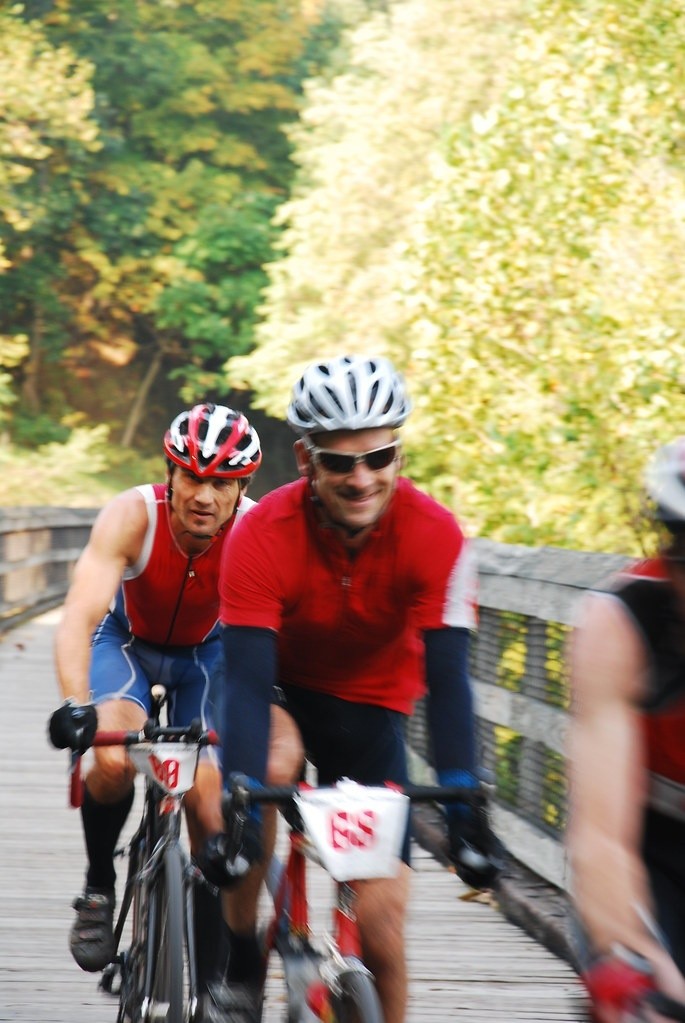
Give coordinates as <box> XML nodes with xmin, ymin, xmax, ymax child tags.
<box><xmin>70</xmin><ymin>883</ymin><xmax>115</xmax><ymax>973</ymax></box>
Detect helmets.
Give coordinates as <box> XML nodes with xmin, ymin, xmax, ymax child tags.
<box><xmin>646</xmin><ymin>436</ymin><xmax>685</xmax><ymax>519</ymax></box>
<box><xmin>162</xmin><ymin>401</ymin><xmax>261</xmax><ymax>479</ymax></box>
<box><xmin>289</xmin><ymin>355</ymin><xmax>407</xmax><ymax>437</ymax></box>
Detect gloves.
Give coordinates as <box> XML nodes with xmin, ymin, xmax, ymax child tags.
<box><xmin>199</xmin><ymin>834</ymin><xmax>248</xmax><ymax>888</ymax></box>
<box><xmin>49</xmin><ymin>702</ymin><xmax>99</xmax><ymax>757</ymax></box>
<box><xmin>450</xmin><ymin>825</ymin><xmax>506</xmax><ymax>890</ymax></box>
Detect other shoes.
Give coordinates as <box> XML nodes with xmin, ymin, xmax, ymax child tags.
<box><xmin>199</xmin><ymin>931</ymin><xmax>264</xmax><ymax>1023</ymax></box>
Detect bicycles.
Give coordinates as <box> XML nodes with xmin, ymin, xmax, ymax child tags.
<box><xmin>212</xmin><ymin>771</ymin><xmax>507</xmax><ymax>1023</ymax></box>
<box><xmin>64</xmin><ymin>707</ymin><xmax>221</xmax><ymax>1023</ymax></box>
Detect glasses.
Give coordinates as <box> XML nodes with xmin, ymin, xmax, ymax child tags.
<box><xmin>304</xmin><ymin>440</ymin><xmax>399</xmax><ymax>474</ymax></box>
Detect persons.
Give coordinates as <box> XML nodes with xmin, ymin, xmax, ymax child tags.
<box><xmin>204</xmin><ymin>352</ymin><xmax>505</xmax><ymax>1022</ymax></box>
<box><xmin>563</xmin><ymin>441</ymin><xmax>685</xmax><ymax>1023</ymax></box>
<box><xmin>55</xmin><ymin>402</ymin><xmax>263</xmax><ymax>971</ymax></box>
